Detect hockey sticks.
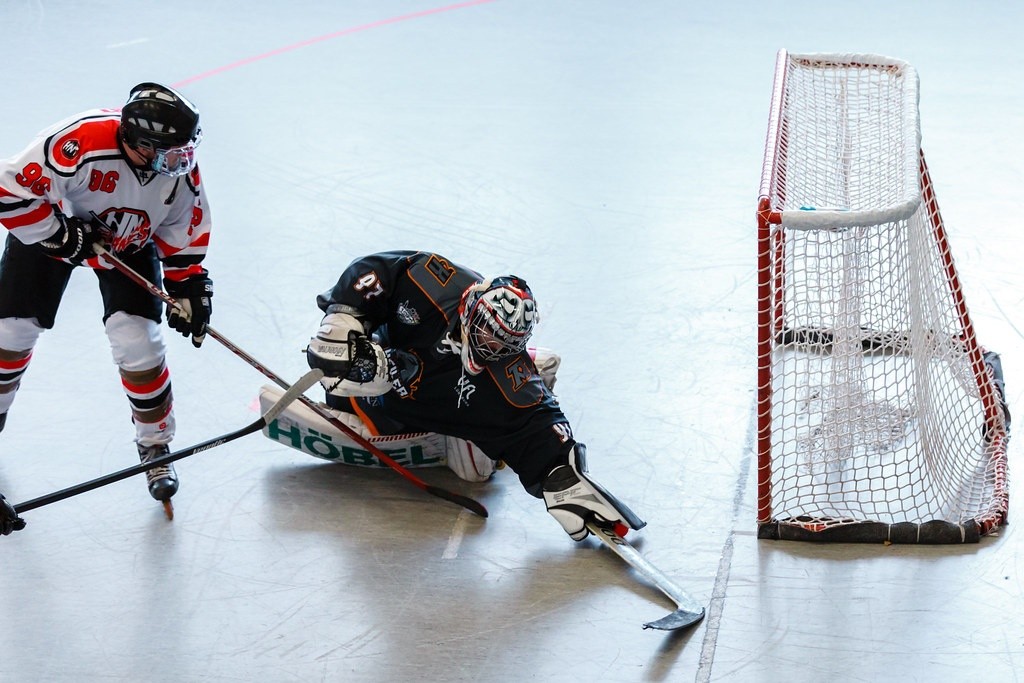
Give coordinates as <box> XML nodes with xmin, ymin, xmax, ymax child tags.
<box><xmin>584</xmin><ymin>519</ymin><xmax>706</xmax><ymax>635</ymax></box>
<box><xmin>89</xmin><ymin>239</ymin><xmax>490</xmax><ymax>523</ymax></box>
<box><xmin>13</xmin><ymin>367</ymin><xmax>326</xmax><ymax>514</ymax></box>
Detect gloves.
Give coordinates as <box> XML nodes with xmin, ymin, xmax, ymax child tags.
<box><xmin>307</xmin><ymin>304</ymin><xmax>394</xmax><ymax>397</ymax></box>
<box><xmin>542</xmin><ymin>442</ymin><xmax>647</xmax><ymax>542</ymax></box>
<box><xmin>35</xmin><ymin>212</ymin><xmax>123</xmax><ymax>270</ymax></box>
<box><xmin>163</xmin><ymin>268</ymin><xmax>213</xmax><ymax>348</ymax></box>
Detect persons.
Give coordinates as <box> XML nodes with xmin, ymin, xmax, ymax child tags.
<box><xmin>0</xmin><ymin>81</ymin><xmax>214</xmax><ymax>502</ymax></box>
<box><xmin>300</xmin><ymin>250</ymin><xmax>646</xmax><ymax>543</ymax></box>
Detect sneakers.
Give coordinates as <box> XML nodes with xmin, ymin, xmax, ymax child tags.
<box><xmin>137</xmin><ymin>442</ymin><xmax>179</xmax><ymax>520</ymax></box>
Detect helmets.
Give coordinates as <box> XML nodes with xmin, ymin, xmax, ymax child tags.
<box><xmin>458</xmin><ymin>275</ymin><xmax>540</xmax><ymax>376</ymax></box>
<box><xmin>121</xmin><ymin>82</ymin><xmax>204</xmax><ymax>154</ymax></box>
